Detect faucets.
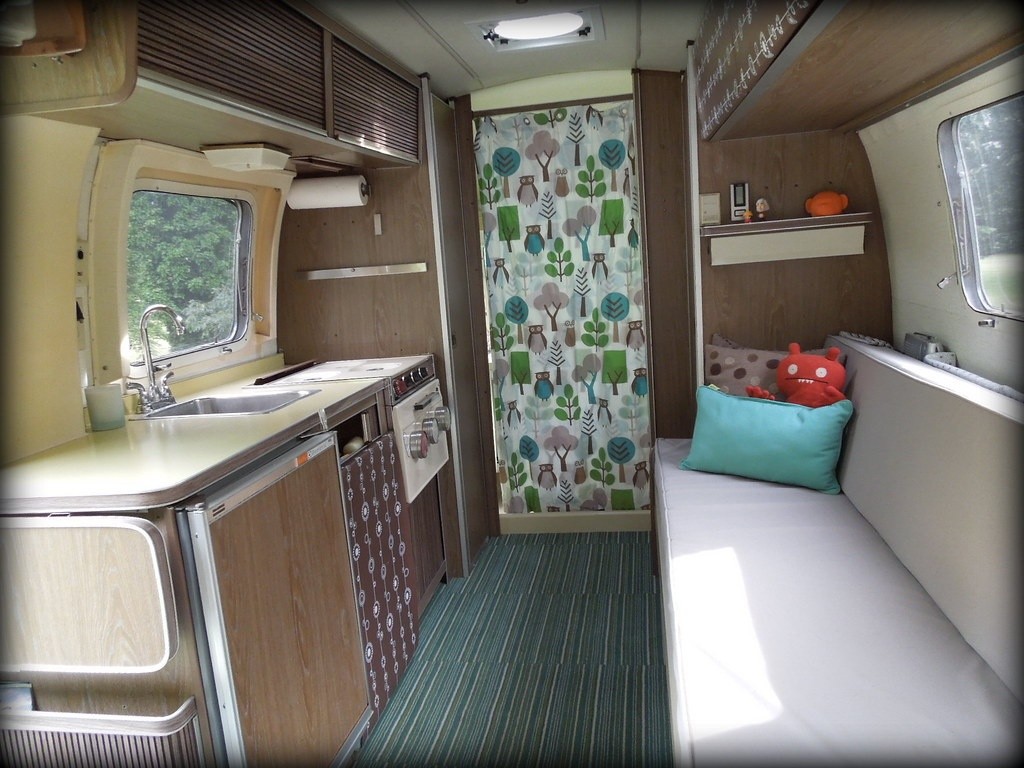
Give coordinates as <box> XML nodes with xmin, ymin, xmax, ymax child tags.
<box><xmin>137</xmin><ymin>304</ymin><xmax>188</xmax><ymax>410</ymax></box>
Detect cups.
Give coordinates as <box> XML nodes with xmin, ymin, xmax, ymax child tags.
<box><xmin>84</xmin><ymin>383</ymin><xmax>125</xmax><ymax>431</ymax></box>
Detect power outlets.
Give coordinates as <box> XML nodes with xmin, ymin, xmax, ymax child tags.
<box><xmin>700</xmin><ymin>193</ymin><xmax>722</xmax><ymax>227</ymax></box>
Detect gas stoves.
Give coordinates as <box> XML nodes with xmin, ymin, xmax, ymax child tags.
<box><xmin>240</xmin><ymin>357</ymin><xmax>450</xmax><ymax>503</ymax></box>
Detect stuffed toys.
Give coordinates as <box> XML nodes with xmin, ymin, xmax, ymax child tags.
<box><xmin>745</xmin><ymin>343</ymin><xmax>847</xmax><ymax>407</ymax></box>
<box><xmin>805</xmin><ymin>191</ymin><xmax>849</xmax><ymax>217</ymax></box>
<box><xmin>743</xmin><ymin>209</ymin><xmax>753</xmax><ymax>223</ymax></box>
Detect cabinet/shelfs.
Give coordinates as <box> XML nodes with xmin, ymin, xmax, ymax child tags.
<box><xmin>340</xmin><ymin>429</ymin><xmax>417</xmax><ymax>750</ymax></box>
<box><xmin>137</xmin><ymin>0</ymin><xmax>327</xmax><ymax>138</ymax></box>
<box><xmin>408</xmin><ymin>474</ymin><xmax>446</xmax><ymax>620</ymax></box>
<box><xmin>183</xmin><ymin>429</ymin><xmax>374</xmax><ymax>768</ymax></box>
<box><xmin>690</xmin><ymin>0</ymin><xmax>820</xmax><ymax>140</ymax></box>
<box><xmin>331</xmin><ymin>33</ymin><xmax>422</xmax><ymax>166</ymax></box>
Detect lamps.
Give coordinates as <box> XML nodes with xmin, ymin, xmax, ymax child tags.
<box><xmin>464</xmin><ymin>3</ymin><xmax>606</xmax><ymax>53</ymax></box>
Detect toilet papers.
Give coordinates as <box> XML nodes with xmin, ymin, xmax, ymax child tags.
<box><xmin>286</xmin><ymin>173</ymin><xmax>368</xmax><ymax>210</ymax></box>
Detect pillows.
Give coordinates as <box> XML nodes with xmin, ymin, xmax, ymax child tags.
<box><xmin>704</xmin><ymin>344</ymin><xmax>848</xmax><ymax>403</ymax></box>
<box><xmin>678</xmin><ymin>383</ymin><xmax>854</xmax><ymax>496</ymax></box>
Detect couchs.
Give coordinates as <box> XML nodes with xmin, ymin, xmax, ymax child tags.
<box><xmin>654</xmin><ymin>333</ymin><xmax>1024</xmax><ymax>768</ymax></box>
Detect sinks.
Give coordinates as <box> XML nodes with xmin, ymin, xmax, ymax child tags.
<box><xmin>130</xmin><ymin>388</ymin><xmax>322</xmax><ymax>416</ymax></box>
